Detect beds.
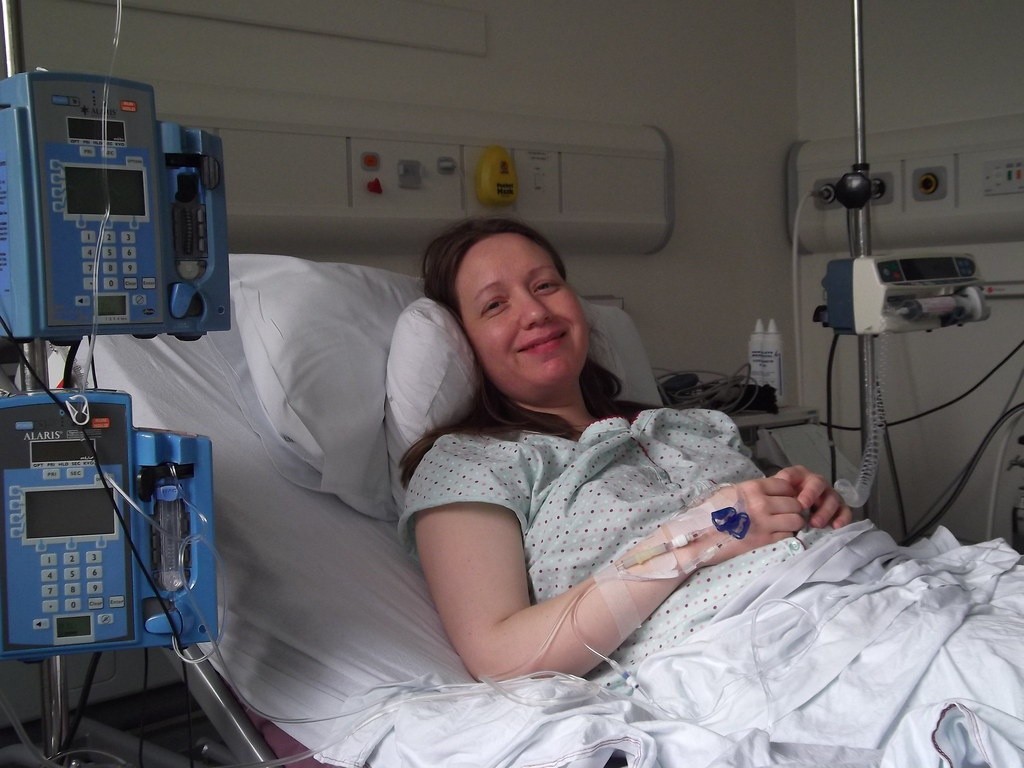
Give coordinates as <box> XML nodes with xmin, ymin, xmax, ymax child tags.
<box><xmin>13</xmin><ymin>255</ymin><xmax>1024</xmax><ymax>768</ymax></box>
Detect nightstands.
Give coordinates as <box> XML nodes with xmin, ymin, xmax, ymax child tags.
<box><xmin>729</xmin><ymin>406</ymin><xmax>818</xmax><ymax>449</ymax></box>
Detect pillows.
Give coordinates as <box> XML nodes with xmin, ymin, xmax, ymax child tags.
<box><xmin>229</xmin><ymin>254</ymin><xmax>662</xmax><ymax>521</ymax></box>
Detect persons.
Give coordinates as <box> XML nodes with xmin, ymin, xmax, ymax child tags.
<box><xmin>396</xmin><ymin>218</ymin><xmax>851</xmax><ymax>693</ymax></box>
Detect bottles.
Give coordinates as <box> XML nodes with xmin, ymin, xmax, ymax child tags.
<box><xmin>763</xmin><ymin>319</ymin><xmax>786</xmax><ymax>406</ymax></box>
<box><xmin>749</xmin><ymin>319</ymin><xmax>764</xmax><ymax>387</ymax></box>
